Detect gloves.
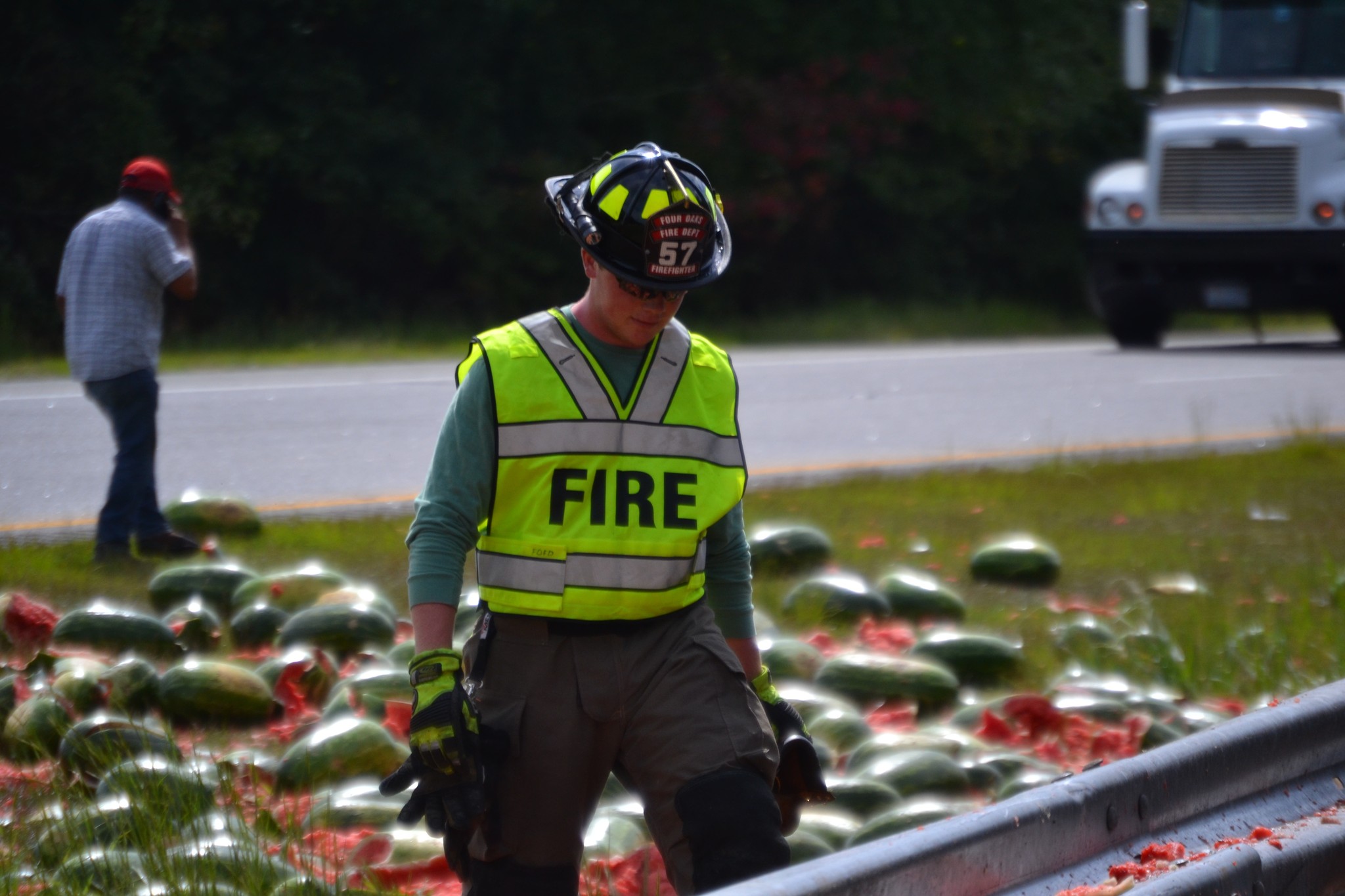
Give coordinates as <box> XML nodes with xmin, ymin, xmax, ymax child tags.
<box><xmin>376</xmin><ymin>644</ymin><xmax>502</xmax><ymax>852</ymax></box>
<box><xmin>751</xmin><ymin>663</ymin><xmax>834</xmax><ymax>839</ymax></box>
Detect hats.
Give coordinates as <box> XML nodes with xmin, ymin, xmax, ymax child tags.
<box><xmin>121</xmin><ymin>157</ymin><xmax>180</xmax><ymax>206</ymax></box>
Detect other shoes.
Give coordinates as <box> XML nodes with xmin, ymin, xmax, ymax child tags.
<box><xmin>97</xmin><ymin>537</ymin><xmax>137</xmax><ymax>560</ymax></box>
<box><xmin>137</xmin><ymin>527</ymin><xmax>199</xmax><ymax>558</ymax></box>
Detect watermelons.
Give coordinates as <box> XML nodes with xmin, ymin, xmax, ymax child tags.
<box><xmin>0</xmin><ymin>499</ymin><xmax>481</xmax><ymax>896</ymax></box>
<box><xmin>583</xmin><ymin>520</ymin><xmax>1230</xmax><ymax>861</ymax></box>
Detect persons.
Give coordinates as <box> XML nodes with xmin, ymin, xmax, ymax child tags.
<box><xmin>54</xmin><ymin>156</ymin><xmax>199</xmax><ymax>572</ymax></box>
<box><xmin>387</xmin><ymin>138</ymin><xmax>811</xmax><ymax>896</ymax></box>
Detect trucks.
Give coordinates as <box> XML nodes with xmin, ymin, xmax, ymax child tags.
<box><xmin>1074</xmin><ymin>0</ymin><xmax>1345</xmax><ymax>351</ymax></box>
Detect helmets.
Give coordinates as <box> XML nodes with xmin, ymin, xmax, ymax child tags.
<box><xmin>546</xmin><ymin>140</ymin><xmax>735</xmax><ymax>291</ymax></box>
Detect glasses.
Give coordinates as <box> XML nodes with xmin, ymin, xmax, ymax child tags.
<box><xmin>615</xmin><ymin>276</ymin><xmax>688</xmax><ymax>303</ymax></box>
